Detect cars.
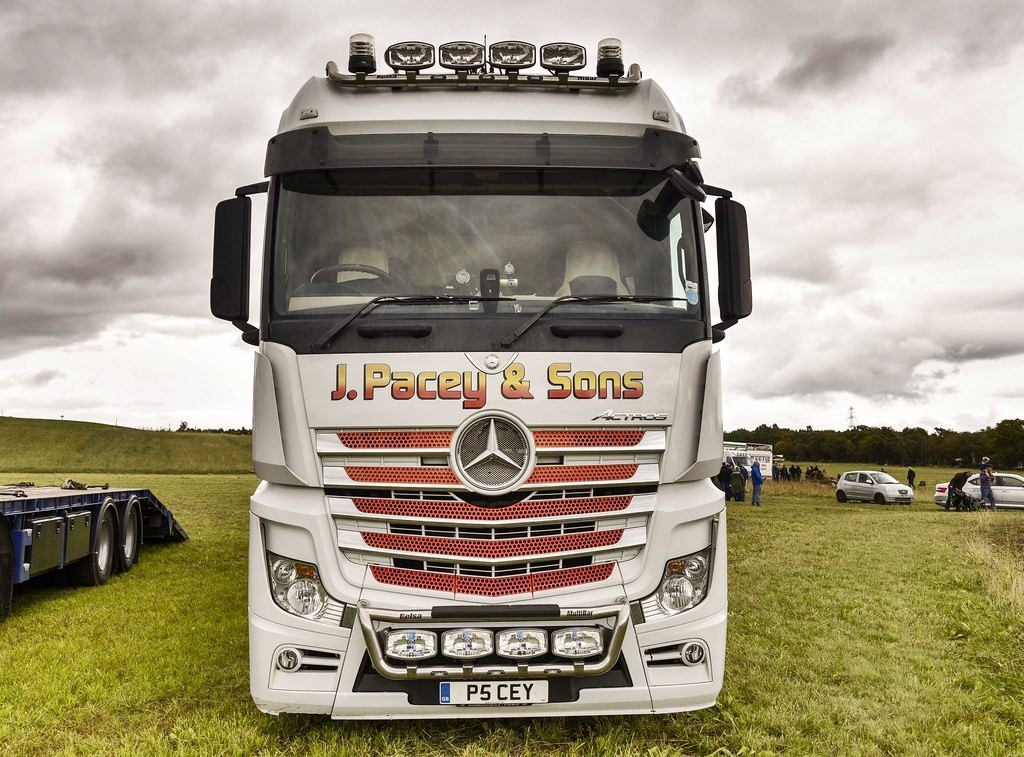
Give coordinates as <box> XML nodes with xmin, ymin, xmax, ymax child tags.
<box><xmin>835</xmin><ymin>470</ymin><xmax>914</xmax><ymax>505</ymax></box>
<box><xmin>933</xmin><ymin>473</ymin><xmax>1024</xmax><ymax>510</ymax></box>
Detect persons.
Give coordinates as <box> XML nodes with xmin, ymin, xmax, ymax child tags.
<box><xmin>979</xmin><ymin>456</ymin><xmax>997</xmax><ymax>511</ymax></box>
<box><xmin>805</xmin><ymin>465</ymin><xmax>828</xmax><ymax>480</ymax></box>
<box><xmin>789</xmin><ymin>465</ymin><xmax>803</xmax><ymax>481</ymax></box>
<box><xmin>772</xmin><ymin>460</ymin><xmax>791</xmax><ymax>481</ymax></box>
<box><xmin>945</xmin><ymin>470</ymin><xmax>973</xmax><ymax>511</ymax></box>
<box><xmin>717</xmin><ymin>461</ymin><xmax>749</xmax><ymax>501</ymax></box>
<box><xmin>750</xmin><ymin>461</ymin><xmax>764</xmax><ymax>506</ymax></box>
<box><xmin>880</xmin><ymin>468</ymin><xmax>885</xmax><ymax>472</ymax></box>
<box><xmin>907</xmin><ymin>467</ymin><xmax>916</xmax><ymax>490</ymax></box>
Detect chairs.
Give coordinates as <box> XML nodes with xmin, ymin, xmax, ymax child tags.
<box><xmin>335</xmin><ymin>247</ymin><xmax>389</xmax><ymax>283</ymax></box>
<box><xmin>555</xmin><ymin>240</ymin><xmax>630</xmax><ymax>297</ymax></box>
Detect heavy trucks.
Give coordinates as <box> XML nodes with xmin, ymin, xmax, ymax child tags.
<box><xmin>212</xmin><ymin>35</ymin><xmax>751</xmax><ymax>718</ymax></box>
